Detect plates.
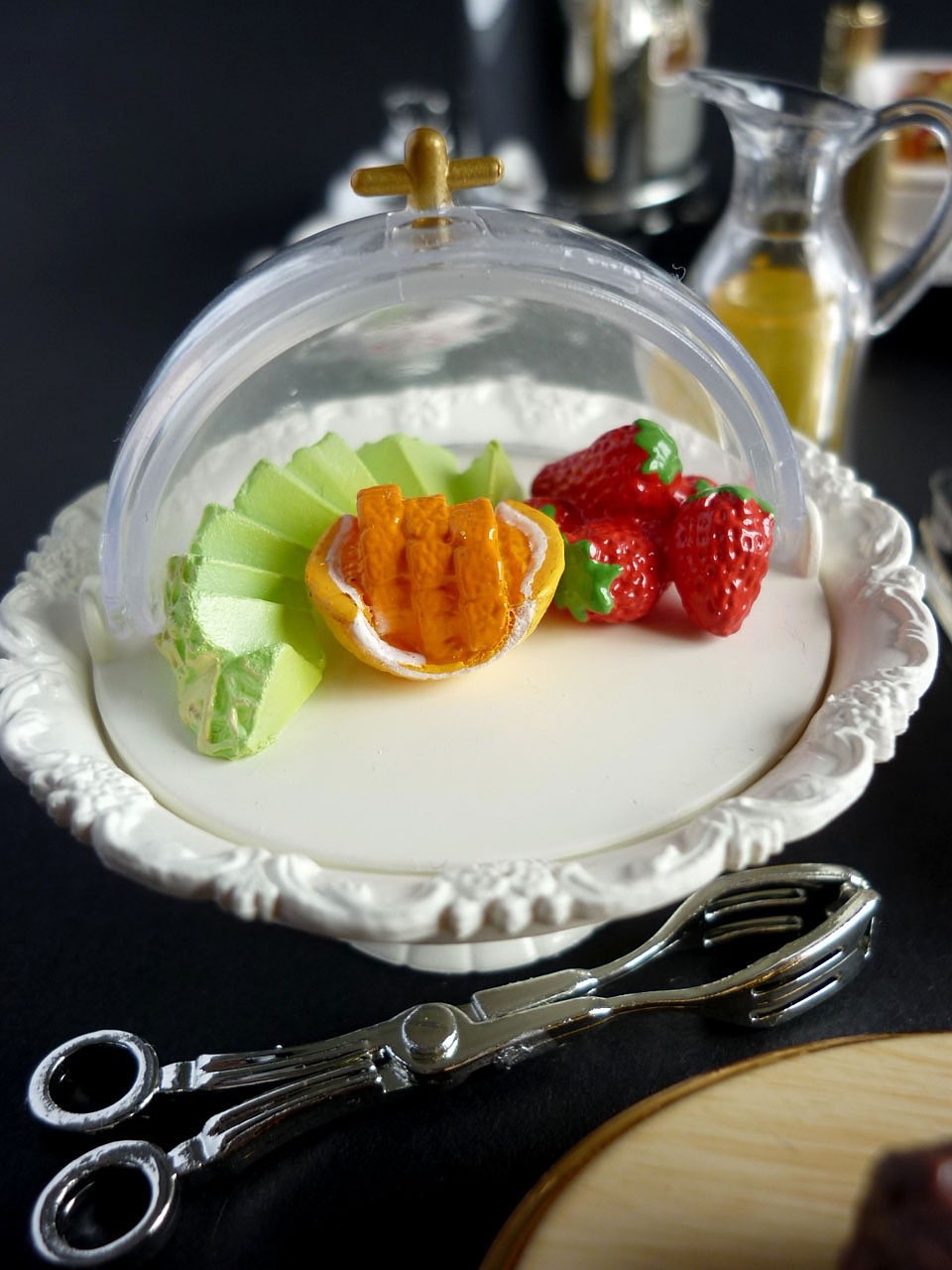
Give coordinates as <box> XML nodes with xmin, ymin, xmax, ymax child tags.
<box><xmin>78</xmin><ymin>448</ymin><xmax>833</xmax><ymax>869</ymax></box>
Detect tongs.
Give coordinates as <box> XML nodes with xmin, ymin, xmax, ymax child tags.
<box><xmin>30</xmin><ymin>865</ymin><xmax>880</xmax><ymax>1270</ymax></box>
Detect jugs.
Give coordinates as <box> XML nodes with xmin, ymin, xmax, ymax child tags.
<box><xmin>680</xmin><ymin>65</ymin><xmax>951</xmax><ymax>451</ymax></box>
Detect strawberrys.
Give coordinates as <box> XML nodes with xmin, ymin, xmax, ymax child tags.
<box><xmin>508</xmin><ymin>421</ymin><xmax>777</xmax><ymax>644</ymax></box>
